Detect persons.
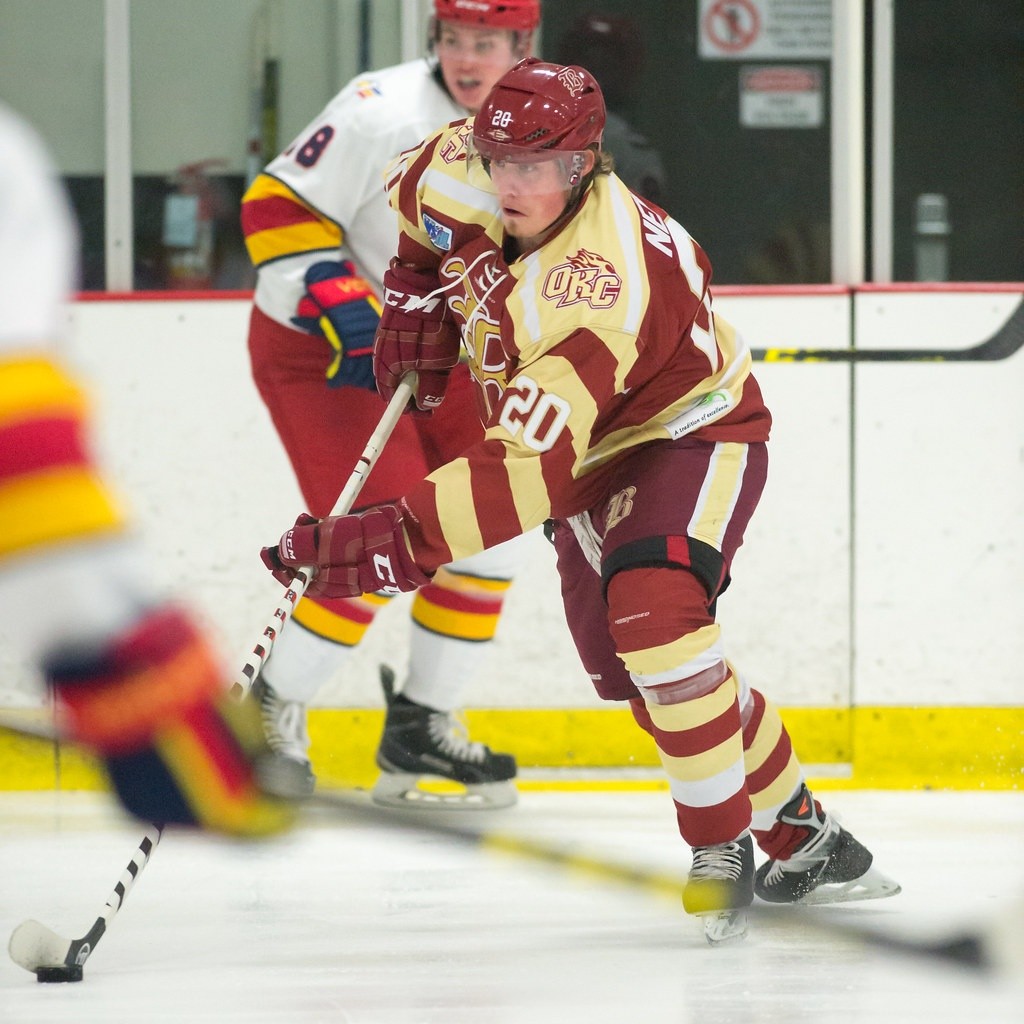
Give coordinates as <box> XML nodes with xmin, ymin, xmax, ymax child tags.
<box><xmin>261</xmin><ymin>57</ymin><xmax>902</xmax><ymax>945</ymax></box>
<box><xmin>0</xmin><ymin>100</ymin><xmax>311</xmax><ymax>838</ymax></box>
<box><xmin>246</xmin><ymin>0</ymin><xmax>540</xmax><ymax>809</ymax></box>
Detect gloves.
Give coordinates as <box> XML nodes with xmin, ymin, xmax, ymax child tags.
<box><xmin>374</xmin><ymin>256</ymin><xmax>462</xmax><ymax>415</ymax></box>
<box><xmin>292</xmin><ymin>260</ymin><xmax>383</xmax><ymax>389</ymax></box>
<box><xmin>260</xmin><ymin>500</ymin><xmax>435</xmax><ymax>598</ymax></box>
<box><xmin>94</xmin><ymin>697</ymin><xmax>300</xmax><ymax>838</ymax></box>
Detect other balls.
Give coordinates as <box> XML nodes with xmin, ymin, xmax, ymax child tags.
<box><xmin>35</xmin><ymin>963</ymin><xmax>84</xmax><ymax>983</ymax></box>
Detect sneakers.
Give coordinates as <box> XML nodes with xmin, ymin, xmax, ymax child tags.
<box><xmin>754</xmin><ymin>812</ymin><xmax>903</xmax><ymax>903</ymax></box>
<box><xmin>680</xmin><ymin>830</ymin><xmax>754</xmax><ymax>941</ymax></box>
<box><xmin>242</xmin><ymin>674</ymin><xmax>320</xmax><ymax>798</ymax></box>
<box><xmin>375</xmin><ymin>665</ymin><xmax>518</xmax><ymax>813</ymax></box>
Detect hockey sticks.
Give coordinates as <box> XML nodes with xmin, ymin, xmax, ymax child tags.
<box><xmin>751</xmin><ymin>295</ymin><xmax>1023</xmax><ymax>362</ymax></box>
<box><xmin>7</xmin><ymin>367</ymin><xmax>417</xmax><ymax>973</ymax></box>
<box><xmin>2</xmin><ymin>717</ymin><xmax>991</xmax><ymax>980</ymax></box>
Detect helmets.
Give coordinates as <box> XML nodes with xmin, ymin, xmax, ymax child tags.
<box><xmin>473</xmin><ymin>57</ymin><xmax>608</xmax><ymax>187</ymax></box>
<box><xmin>434</xmin><ymin>0</ymin><xmax>541</xmax><ymax>53</ymax></box>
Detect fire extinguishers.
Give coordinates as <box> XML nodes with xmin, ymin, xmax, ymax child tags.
<box><xmin>160</xmin><ymin>157</ymin><xmax>232</xmax><ymax>289</ymax></box>
